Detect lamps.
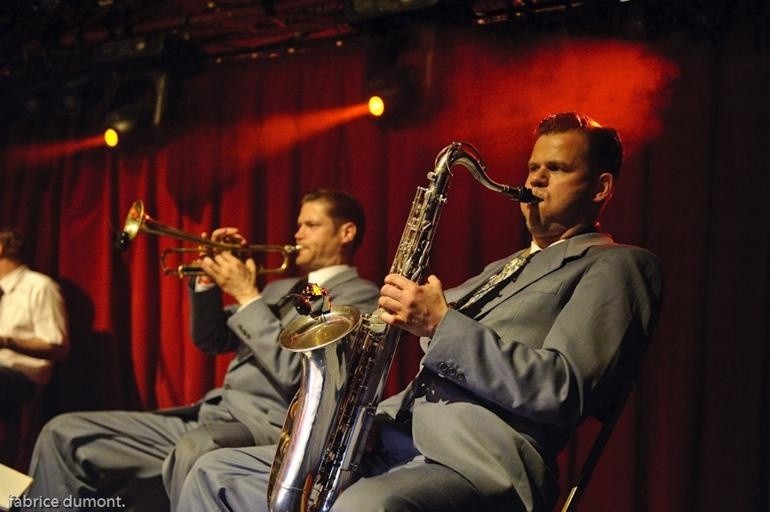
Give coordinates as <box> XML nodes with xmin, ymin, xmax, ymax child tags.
<box><xmin>368</xmin><ymin>82</ymin><xmax>416</xmax><ymax>126</ymax></box>
<box><xmin>103</xmin><ymin>118</ymin><xmax>153</xmax><ymax>154</ymax></box>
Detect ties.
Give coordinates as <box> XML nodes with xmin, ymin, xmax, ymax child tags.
<box><xmin>446</xmin><ymin>247</ymin><xmax>540</xmax><ymax>311</ymax></box>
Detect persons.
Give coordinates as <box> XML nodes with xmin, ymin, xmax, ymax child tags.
<box><xmin>0</xmin><ymin>225</ymin><xmax>72</xmax><ymax>469</ymax></box>
<box><xmin>20</xmin><ymin>189</ymin><xmax>383</xmax><ymax>512</ymax></box>
<box><xmin>179</xmin><ymin>111</ymin><xmax>665</xmax><ymax>512</ymax></box>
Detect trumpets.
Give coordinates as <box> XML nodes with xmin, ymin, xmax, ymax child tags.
<box><xmin>119</xmin><ymin>200</ymin><xmax>307</xmax><ymax>279</ymax></box>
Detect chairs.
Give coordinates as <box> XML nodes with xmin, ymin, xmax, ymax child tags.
<box><xmin>546</xmin><ymin>299</ymin><xmax>663</xmax><ymax>512</ymax></box>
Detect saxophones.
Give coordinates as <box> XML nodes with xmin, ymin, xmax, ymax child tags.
<box><xmin>266</xmin><ymin>139</ymin><xmax>543</xmax><ymax>512</ymax></box>
